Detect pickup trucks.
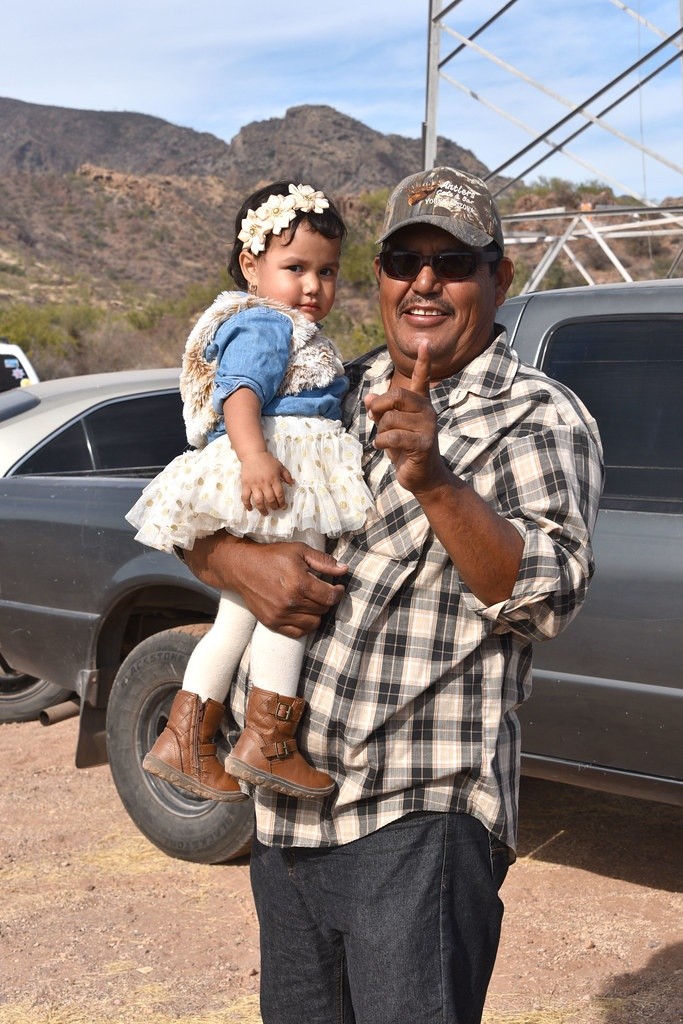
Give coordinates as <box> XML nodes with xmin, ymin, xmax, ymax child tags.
<box><xmin>1</xmin><ymin>279</ymin><xmax>683</xmax><ymax>865</ymax></box>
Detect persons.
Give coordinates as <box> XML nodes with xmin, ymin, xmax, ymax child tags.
<box><xmin>123</xmin><ymin>179</ymin><xmax>376</xmax><ymax>802</ymax></box>
<box><xmin>173</xmin><ymin>167</ymin><xmax>606</xmax><ymax>1024</ymax></box>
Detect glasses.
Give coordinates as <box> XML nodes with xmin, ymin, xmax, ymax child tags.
<box><xmin>378</xmin><ymin>252</ymin><xmax>496</xmax><ymax>282</ymax></box>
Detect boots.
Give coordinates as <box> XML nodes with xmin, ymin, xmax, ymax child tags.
<box><xmin>224</xmin><ymin>687</ymin><xmax>336</xmax><ymax>799</ymax></box>
<box><xmin>142</xmin><ymin>690</ymin><xmax>247</xmax><ymax>803</ymax></box>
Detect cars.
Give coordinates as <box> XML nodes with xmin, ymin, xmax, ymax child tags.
<box><xmin>0</xmin><ymin>367</ymin><xmax>196</xmax><ymax>725</ymax></box>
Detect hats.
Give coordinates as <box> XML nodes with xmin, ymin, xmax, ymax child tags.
<box><xmin>374</xmin><ymin>166</ymin><xmax>504</xmax><ymax>255</ymax></box>
